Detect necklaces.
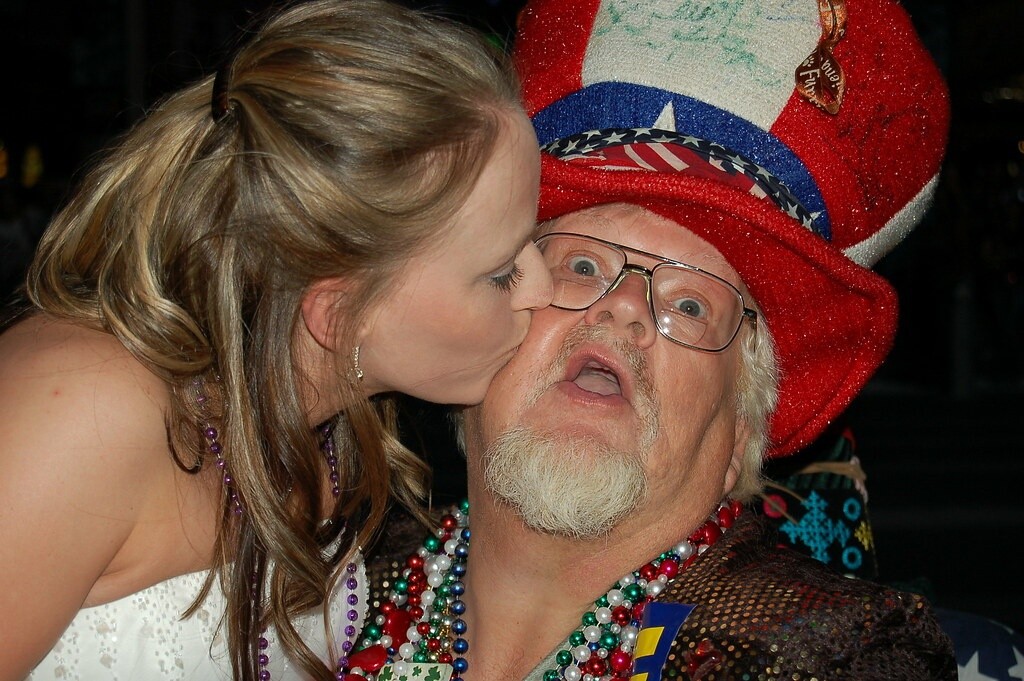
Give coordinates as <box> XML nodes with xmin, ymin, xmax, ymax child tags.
<box><xmin>192</xmin><ymin>378</ymin><xmax>358</xmax><ymax>681</ymax></box>
<box><xmin>342</xmin><ymin>499</ymin><xmax>744</xmax><ymax>681</ymax></box>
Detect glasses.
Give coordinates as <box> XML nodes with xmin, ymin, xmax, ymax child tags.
<box><xmin>535</xmin><ymin>231</ymin><xmax>758</xmax><ymax>353</ymax></box>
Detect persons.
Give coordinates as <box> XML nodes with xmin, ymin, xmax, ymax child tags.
<box><xmin>362</xmin><ymin>0</ymin><xmax>959</xmax><ymax>681</ymax></box>
<box><xmin>0</xmin><ymin>0</ymin><xmax>556</xmax><ymax>681</ymax></box>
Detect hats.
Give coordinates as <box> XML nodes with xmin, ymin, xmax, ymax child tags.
<box><xmin>513</xmin><ymin>0</ymin><xmax>948</xmax><ymax>459</ymax></box>
